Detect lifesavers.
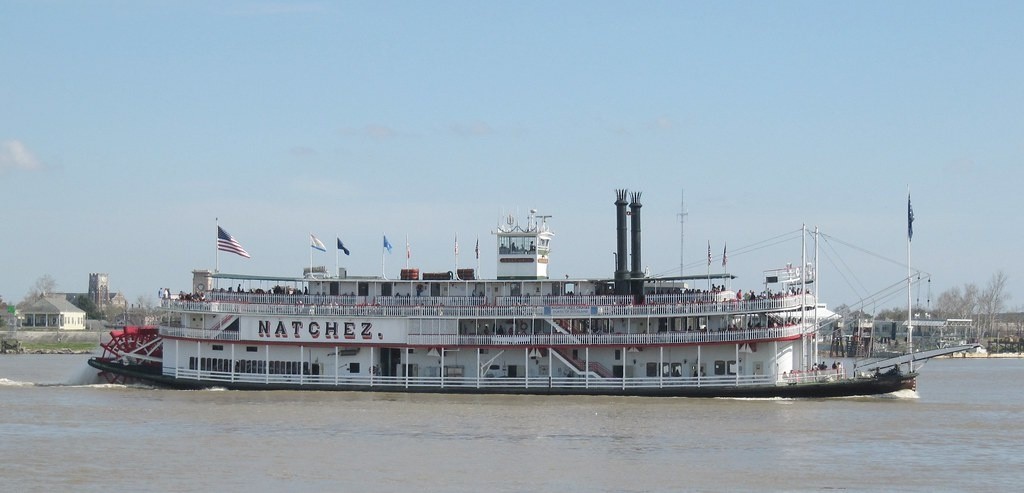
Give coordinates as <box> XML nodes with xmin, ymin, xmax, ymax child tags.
<box><xmin>520</xmin><ymin>322</ymin><xmax>527</xmax><ymax>330</ymax></box>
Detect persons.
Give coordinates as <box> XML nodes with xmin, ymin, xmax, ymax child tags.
<box><xmin>162</xmin><ymin>281</ymin><xmax>846</xmax><ymax>385</ymax></box>
<box><xmin>500</xmin><ymin>241</ymin><xmax>537</xmax><ymax>253</ymax></box>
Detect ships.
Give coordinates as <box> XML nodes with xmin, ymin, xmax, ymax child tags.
<box><xmin>87</xmin><ymin>188</ymin><xmax>984</xmax><ymax>398</ymax></box>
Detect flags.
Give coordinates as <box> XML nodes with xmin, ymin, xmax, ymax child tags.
<box><xmin>406</xmin><ymin>244</ymin><xmax>411</xmax><ymax>258</ymax></box>
<box><xmin>907</xmin><ymin>196</ymin><xmax>916</xmax><ymax>240</ymax></box>
<box><xmin>217</xmin><ymin>226</ymin><xmax>250</xmax><ymax>258</ymax></box>
<box><xmin>475</xmin><ymin>243</ymin><xmax>481</xmax><ymax>258</ymax></box>
<box><xmin>310</xmin><ymin>234</ymin><xmax>327</xmax><ymax>252</ymax></box>
<box><xmin>456</xmin><ymin>241</ymin><xmax>459</xmax><ymax>255</ymax></box>
<box><xmin>384</xmin><ymin>237</ymin><xmax>394</xmax><ymax>251</ymax></box>
<box><xmin>708</xmin><ymin>246</ymin><xmax>712</xmax><ymax>265</ymax></box>
<box><xmin>724</xmin><ymin>246</ymin><xmax>728</xmax><ymax>266</ymax></box>
<box><xmin>337</xmin><ymin>240</ymin><xmax>350</xmax><ymax>255</ymax></box>
